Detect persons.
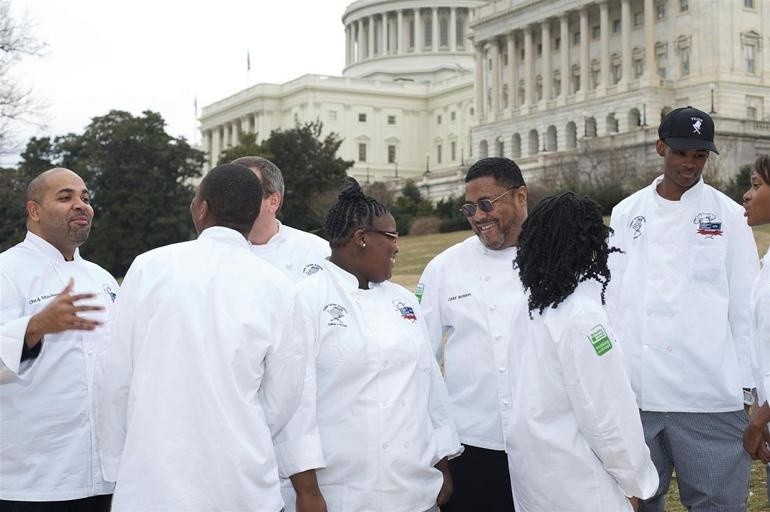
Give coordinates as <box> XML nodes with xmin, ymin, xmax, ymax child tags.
<box><xmin>0</xmin><ymin>168</ymin><xmax>122</xmax><ymax>511</ymax></box>
<box><xmin>231</xmin><ymin>154</ymin><xmax>332</xmax><ymax>285</ymax></box>
<box><xmin>499</xmin><ymin>190</ymin><xmax>662</xmax><ymax>512</ymax></box>
<box><xmin>603</xmin><ymin>106</ymin><xmax>762</xmax><ymax>512</ymax></box>
<box><xmin>268</xmin><ymin>185</ymin><xmax>466</xmax><ymax>512</ymax></box>
<box><xmin>90</xmin><ymin>163</ymin><xmax>329</xmax><ymax>512</ymax></box>
<box><xmin>413</xmin><ymin>157</ymin><xmax>533</xmax><ymax>512</ymax></box>
<box><xmin>741</xmin><ymin>151</ymin><xmax>769</xmax><ymax>466</ymax></box>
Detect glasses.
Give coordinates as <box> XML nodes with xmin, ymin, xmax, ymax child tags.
<box><xmin>459</xmin><ymin>188</ymin><xmax>515</xmax><ymax>218</ymax></box>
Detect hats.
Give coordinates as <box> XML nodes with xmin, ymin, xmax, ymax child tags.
<box><xmin>657</xmin><ymin>105</ymin><xmax>720</xmax><ymax>156</ymax></box>
